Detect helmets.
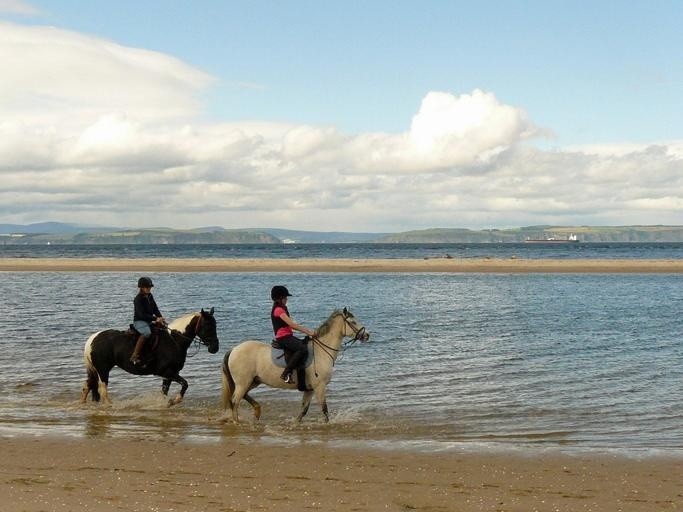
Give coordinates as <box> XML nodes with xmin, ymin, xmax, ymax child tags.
<box><xmin>271</xmin><ymin>286</ymin><xmax>292</xmax><ymax>300</ymax></box>
<box><xmin>137</xmin><ymin>277</ymin><xmax>153</xmax><ymax>288</ymax></box>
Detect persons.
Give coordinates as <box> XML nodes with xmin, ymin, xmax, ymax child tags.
<box><xmin>129</xmin><ymin>275</ymin><xmax>167</xmax><ymax>370</ymax></box>
<box><xmin>270</xmin><ymin>285</ymin><xmax>319</xmax><ymax>385</ymax></box>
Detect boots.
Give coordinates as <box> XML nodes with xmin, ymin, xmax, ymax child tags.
<box><xmin>130</xmin><ymin>335</ymin><xmax>149</xmax><ymax>365</ymax></box>
<box><xmin>280</xmin><ymin>350</ymin><xmax>303</xmax><ymax>384</ymax></box>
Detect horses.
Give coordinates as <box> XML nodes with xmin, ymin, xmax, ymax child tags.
<box><xmin>221</xmin><ymin>306</ymin><xmax>370</xmax><ymax>423</ymax></box>
<box><xmin>80</xmin><ymin>306</ymin><xmax>219</xmax><ymax>407</ymax></box>
